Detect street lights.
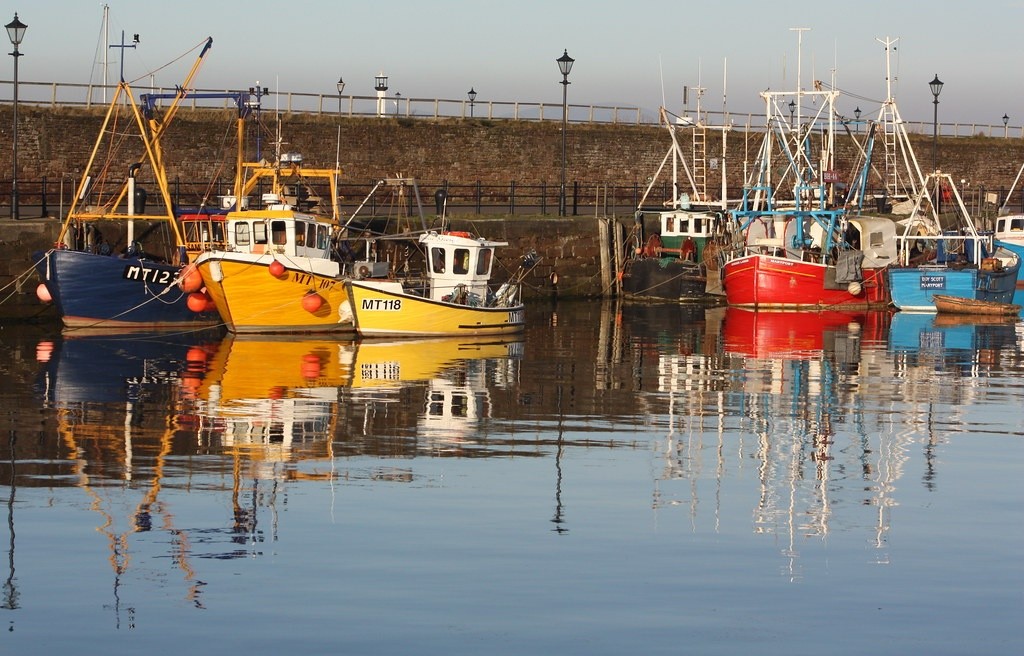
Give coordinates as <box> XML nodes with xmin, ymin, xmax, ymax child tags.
<box><xmin>467</xmin><ymin>86</ymin><xmax>477</xmax><ymax>120</ymax></box>
<box><xmin>2</xmin><ymin>12</ymin><xmax>29</xmax><ymax>218</ymax></box>
<box><xmin>788</xmin><ymin>100</ymin><xmax>798</xmax><ymax>129</ymax></box>
<box><xmin>337</xmin><ymin>77</ymin><xmax>345</xmax><ymax>114</ymax></box>
<box><xmin>929</xmin><ymin>73</ymin><xmax>945</xmax><ymax>211</ymax></box>
<box><xmin>555</xmin><ymin>48</ymin><xmax>576</xmax><ymax>214</ymax></box>
<box><xmin>853</xmin><ymin>105</ymin><xmax>862</xmax><ymax>133</ymax></box>
<box><xmin>1002</xmin><ymin>112</ymin><xmax>1010</xmax><ymax>138</ymax></box>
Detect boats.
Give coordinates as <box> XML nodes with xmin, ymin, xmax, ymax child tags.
<box><xmin>30</xmin><ymin>28</ymin><xmax>540</xmax><ymax>337</ymax></box>
<box><xmin>659</xmin><ymin>26</ymin><xmax>1024</xmax><ymax>313</ymax></box>
